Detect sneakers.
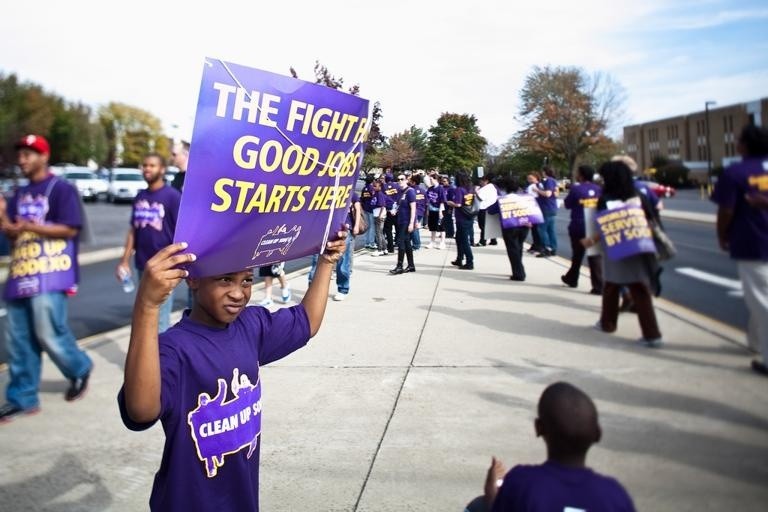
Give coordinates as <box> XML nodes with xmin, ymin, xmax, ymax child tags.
<box><xmin>425</xmin><ymin>242</ymin><xmax>447</xmax><ymax>250</ymax></box>
<box><xmin>476</xmin><ymin>240</ymin><xmax>497</xmax><ymax>246</ymax></box>
<box><xmin>561</xmin><ymin>275</ymin><xmax>663</xmax><ymax>347</ymax></box>
<box><xmin>257</xmin><ymin>286</ymin><xmax>291</xmax><ymax>308</ymax></box>
<box><xmin>364</xmin><ymin>243</ymin><xmax>394</xmax><ymax>257</ymax></box>
<box><xmin>389</xmin><ymin>264</ymin><xmax>415</xmax><ymax>274</ymax></box>
<box><xmin>451</xmin><ymin>260</ymin><xmax>474</xmax><ymax>270</ymax></box>
<box><xmin>508</xmin><ymin>244</ymin><xmax>557</xmax><ymax>282</ymax></box>
<box><xmin>333</xmin><ymin>289</ymin><xmax>351</xmax><ymax>301</ymax></box>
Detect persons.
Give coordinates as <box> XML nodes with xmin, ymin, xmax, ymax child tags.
<box><xmin>169</xmin><ymin>141</ymin><xmax>190</xmax><ymax>192</ymax></box>
<box><xmin>1</xmin><ymin>134</ymin><xmax>93</xmax><ymax>421</ymax></box>
<box><xmin>709</xmin><ymin>125</ymin><xmax>767</xmax><ymax>375</ymax></box>
<box><xmin>561</xmin><ymin>155</ymin><xmax>664</xmax><ymax>346</ymax></box>
<box><xmin>308</xmin><ymin>192</ymin><xmax>360</xmax><ymax>301</ymax></box>
<box><xmin>113</xmin><ymin>152</ymin><xmax>182</xmax><ymax>334</ymax></box>
<box><xmin>259</xmin><ymin>261</ymin><xmax>291</xmax><ymax>307</ymax></box>
<box><xmin>465</xmin><ymin>382</ymin><xmax>635</xmax><ymax>512</ymax></box>
<box><xmin>360</xmin><ymin>167</ymin><xmax>560</xmax><ymax>281</ymax></box>
<box><xmin>118</xmin><ymin>222</ymin><xmax>351</xmax><ymax>512</ymax></box>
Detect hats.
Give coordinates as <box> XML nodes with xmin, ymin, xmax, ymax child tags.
<box><xmin>14</xmin><ymin>134</ymin><xmax>51</xmax><ymax>157</ymax></box>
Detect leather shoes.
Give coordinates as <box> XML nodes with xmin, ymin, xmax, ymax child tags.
<box><xmin>65</xmin><ymin>372</ymin><xmax>90</xmax><ymax>400</ymax></box>
<box><xmin>0</xmin><ymin>402</ymin><xmax>24</xmax><ymax>419</ymax></box>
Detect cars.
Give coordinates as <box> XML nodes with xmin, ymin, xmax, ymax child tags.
<box><xmin>643</xmin><ymin>180</ymin><xmax>676</xmax><ymax>198</ymax></box>
<box><xmin>0</xmin><ymin>162</ymin><xmax>182</xmax><ymax>209</ymax></box>
<box><xmin>558</xmin><ymin>184</ymin><xmax>565</xmax><ymax>192</ymax></box>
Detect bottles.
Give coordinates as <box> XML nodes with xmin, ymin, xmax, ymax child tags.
<box><xmin>392</xmin><ymin>202</ymin><xmax>398</xmax><ymax>213</ymax></box>
<box><xmin>119</xmin><ymin>267</ymin><xmax>135</xmax><ymax>294</ymax></box>
<box><xmin>438</xmin><ymin>218</ymin><xmax>442</xmax><ymax>226</ymax></box>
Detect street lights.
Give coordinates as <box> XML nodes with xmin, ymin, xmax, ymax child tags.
<box><xmin>705</xmin><ymin>100</ymin><xmax>717</xmax><ymax>194</ymax></box>
